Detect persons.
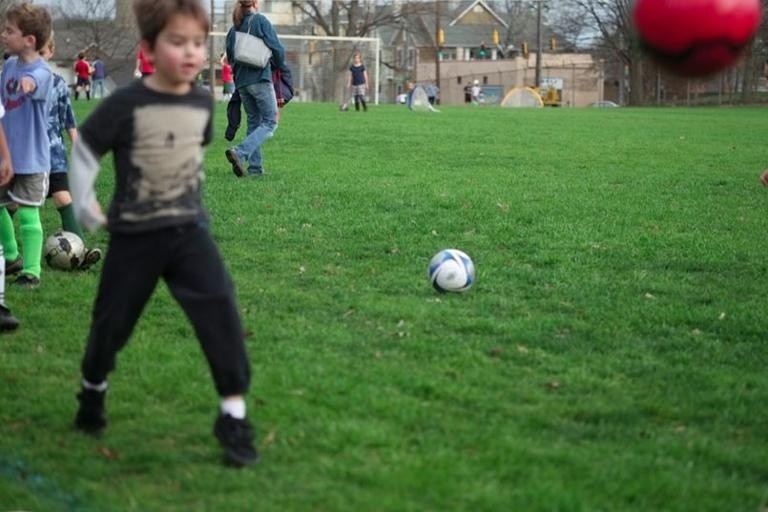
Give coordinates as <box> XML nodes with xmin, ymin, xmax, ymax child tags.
<box><xmin>134</xmin><ymin>46</ymin><xmax>156</xmax><ymax>79</ymax></box>
<box><xmin>65</xmin><ymin>1</ymin><xmax>259</xmax><ymax>467</ymax></box>
<box><xmin>395</xmin><ymin>79</ymin><xmax>483</xmax><ymax>106</ymax></box>
<box><xmin>348</xmin><ymin>53</ymin><xmax>368</xmax><ymax>112</ymax></box>
<box><xmin>0</xmin><ymin>0</ymin><xmax>109</xmax><ymax>332</ymax></box>
<box><xmin>220</xmin><ymin>49</ymin><xmax>234</xmax><ymax>103</ymax></box>
<box><xmin>225</xmin><ymin>0</ymin><xmax>285</xmax><ymax>177</ymax></box>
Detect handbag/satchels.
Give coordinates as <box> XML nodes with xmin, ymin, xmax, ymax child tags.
<box><xmin>234</xmin><ymin>31</ymin><xmax>272</xmax><ymax>68</ymax></box>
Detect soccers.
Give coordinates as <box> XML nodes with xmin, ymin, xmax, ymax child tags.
<box><xmin>45</xmin><ymin>231</ymin><xmax>84</xmax><ymax>268</ymax></box>
<box><xmin>428</xmin><ymin>248</ymin><xmax>476</xmax><ymax>294</ymax></box>
<box><xmin>340</xmin><ymin>103</ymin><xmax>349</xmax><ymax>111</ymax></box>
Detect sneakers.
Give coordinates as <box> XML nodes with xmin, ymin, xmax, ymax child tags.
<box><xmin>81</xmin><ymin>248</ymin><xmax>102</xmax><ymax>269</ymax></box>
<box><xmin>0</xmin><ymin>302</ymin><xmax>19</xmax><ymax>329</ymax></box>
<box><xmin>75</xmin><ymin>388</ymin><xmax>106</xmax><ymax>435</ymax></box>
<box><xmin>5</xmin><ymin>256</ymin><xmax>40</xmax><ymax>286</ymax></box>
<box><xmin>225</xmin><ymin>149</ymin><xmax>243</xmax><ymax>177</ymax></box>
<box><xmin>214</xmin><ymin>414</ymin><xmax>259</xmax><ymax>466</ymax></box>
